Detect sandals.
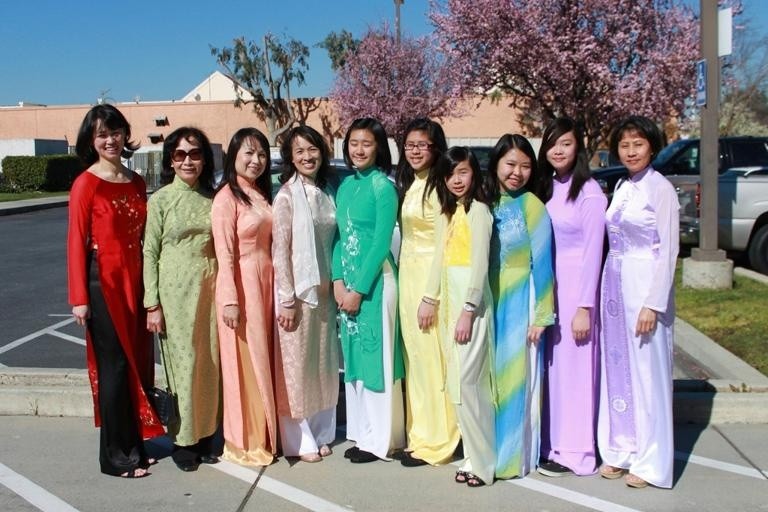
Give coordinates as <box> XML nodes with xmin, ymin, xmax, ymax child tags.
<box><xmin>116</xmin><ymin>467</ymin><xmax>146</xmax><ymax>478</ymax></box>
<box><xmin>139</xmin><ymin>455</ymin><xmax>155</xmax><ymax>465</ymax></box>
<box><xmin>301</xmin><ymin>453</ymin><xmax>320</xmax><ymax>463</ymax></box>
<box><xmin>392</xmin><ymin>447</ymin><xmax>414</xmax><ymax>460</ymax></box>
<box><xmin>401</xmin><ymin>452</ymin><xmax>428</xmax><ymax>467</ymax></box>
<box><xmin>600</xmin><ymin>465</ymin><xmax>625</xmax><ymax>479</ymax></box>
<box><xmin>319</xmin><ymin>444</ymin><xmax>331</xmax><ymax>456</ymax></box>
<box><xmin>626</xmin><ymin>473</ymin><xmax>650</xmax><ymax>488</ymax></box>
<box><xmin>467</xmin><ymin>474</ymin><xmax>484</xmax><ymax>486</ymax></box>
<box><xmin>455</xmin><ymin>471</ymin><xmax>469</xmax><ymax>482</ymax></box>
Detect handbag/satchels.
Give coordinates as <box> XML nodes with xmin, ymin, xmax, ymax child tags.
<box><xmin>145</xmin><ymin>330</ymin><xmax>177</xmax><ymax>425</ymax></box>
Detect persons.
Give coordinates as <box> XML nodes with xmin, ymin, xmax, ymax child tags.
<box><xmin>437</xmin><ymin>146</ymin><xmax>496</xmax><ymax>488</ymax></box>
<box><xmin>535</xmin><ymin>116</ymin><xmax>607</xmax><ymax>476</ymax></box>
<box><xmin>270</xmin><ymin>126</ymin><xmax>338</xmax><ymax>463</ymax></box>
<box><xmin>596</xmin><ymin>114</ymin><xmax>680</xmax><ymax>490</ymax></box>
<box><xmin>488</xmin><ymin>133</ymin><xmax>557</xmax><ymax>480</ymax></box>
<box><xmin>68</xmin><ymin>104</ymin><xmax>161</xmax><ymax>478</ymax></box>
<box><xmin>398</xmin><ymin>117</ymin><xmax>461</xmax><ymax>467</ymax></box>
<box><xmin>143</xmin><ymin>126</ymin><xmax>220</xmax><ymax>473</ymax></box>
<box><xmin>211</xmin><ymin>127</ymin><xmax>277</xmax><ymax>467</ymax></box>
<box><xmin>331</xmin><ymin>117</ymin><xmax>405</xmax><ymax>463</ymax></box>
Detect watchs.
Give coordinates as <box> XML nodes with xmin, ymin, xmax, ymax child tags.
<box><xmin>463</xmin><ymin>304</ymin><xmax>475</xmax><ymax>312</ymax></box>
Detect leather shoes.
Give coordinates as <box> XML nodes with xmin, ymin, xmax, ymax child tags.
<box><xmin>344</xmin><ymin>447</ymin><xmax>360</xmax><ymax>459</ymax></box>
<box><xmin>175</xmin><ymin>458</ymin><xmax>198</xmax><ymax>472</ymax></box>
<box><xmin>351</xmin><ymin>451</ymin><xmax>378</xmax><ymax>463</ymax></box>
<box><xmin>199</xmin><ymin>452</ymin><xmax>218</xmax><ymax>464</ymax></box>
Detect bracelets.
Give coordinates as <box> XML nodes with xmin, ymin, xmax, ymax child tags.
<box><xmin>281</xmin><ymin>300</ymin><xmax>296</xmax><ymax>308</ymax></box>
<box><xmin>421</xmin><ymin>297</ymin><xmax>439</xmax><ymax>305</ymax></box>
<box><xmin>147</xmin><ymin>305</ymin><xmax>161</xmax><ymax>313</ymax></box>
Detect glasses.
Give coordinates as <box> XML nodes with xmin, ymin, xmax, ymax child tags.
<box><xmin>170</xmin><ymin>148</ymin><xmax>204</xmax><ymax>162</ymax></box>
<box><xmin>404</xmin><ymin>143</ymin><xmax>433</xmax><ymax>150</ymax></box>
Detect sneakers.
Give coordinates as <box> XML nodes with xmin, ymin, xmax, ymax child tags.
<box><xmin>537</xmin><ymin>462</ymin><xmax>574</xmax><ymax>477</ymax></box>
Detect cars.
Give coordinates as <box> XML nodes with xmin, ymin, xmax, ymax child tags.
<box><xmin>663</xmin><ymin>167</ymin><xmax>768</xmax><ymax>275</ymax></box>
<box><xmin>203</xmin><ymin>160</ymin><xmax>402</xmax><ymax>197</ymax></box>
<box><xmin>593</xmin><ymin>136</ymin><xmax>768</xmax><ymax>274</ymax></box>
